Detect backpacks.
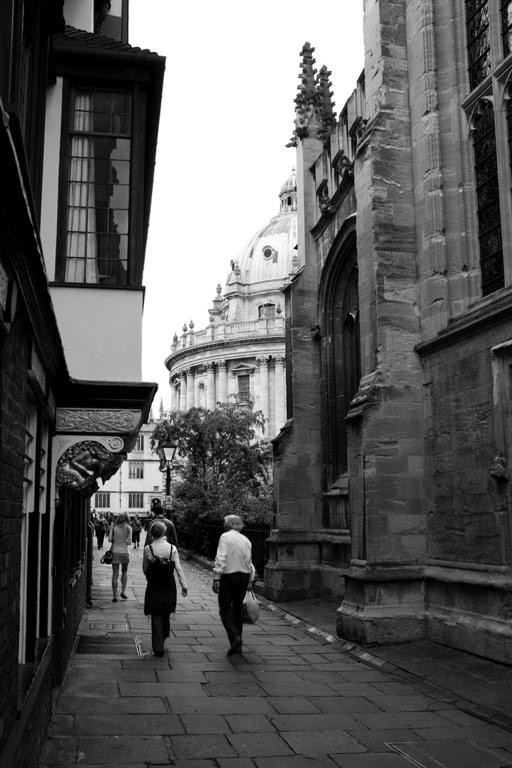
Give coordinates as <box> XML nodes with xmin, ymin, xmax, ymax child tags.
<box><xmin>149</xmin><ymin>543</ymin><xmax>175</xmax><ymax>596</ymax></box>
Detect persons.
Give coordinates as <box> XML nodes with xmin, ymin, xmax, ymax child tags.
<box><xmin>91</xmin><ymin>509</ymin><xmax>156</xmax><ymax>549</ymax></box>
<box><xmin>108</xmin><ymin>511</ymin><xmax>133</xmax><ymax>602</ymax></box>
<box><xmin>142</xmin><ymin>505</ymin><xmax>179</xmax><ymax>549</ymax></box>
<box><xmin>212</xmin><ymin>513</ymin><xmax>255</xmax><ymax>655</ymax></box>
<box><xmin>142</xmin><ymin>520</ymin><xmax>188</xmax><ymax>656</ymax></box>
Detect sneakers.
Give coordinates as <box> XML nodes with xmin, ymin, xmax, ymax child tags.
<box><xmin>226</xmin><ymin>635</ymin><xmax>242</xmax><ymax>656</ymax></box>
<box><xmin>112</xmin><ymin>592</ymin><xmax>127</xmax><ymax>602</ymax></box>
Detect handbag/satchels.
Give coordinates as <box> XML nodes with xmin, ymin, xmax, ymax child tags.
<box><xmin>103</xmin><ymin>550</ymin><xmax>114</xmax><ymax>564</ymax></box>
